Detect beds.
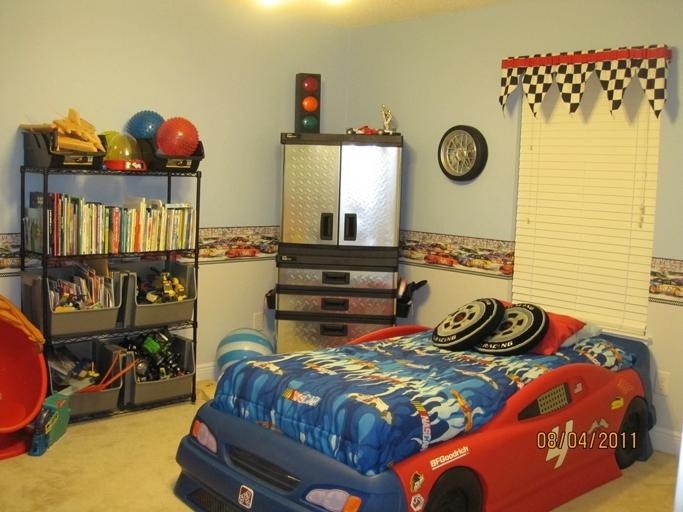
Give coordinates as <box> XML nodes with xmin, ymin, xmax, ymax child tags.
<box><xmin>177</xmin><ymin>323</ymin><xmax>655</xmax><ymax>507</ymax></box>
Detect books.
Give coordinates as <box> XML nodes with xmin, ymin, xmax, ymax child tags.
<box><xmin>23</xmin><ymin>190</ymin><xmax>197</xmax><ymax>257</ymax></box>
<box><xmin>44</xmin><ymin>257</ymin><xmax>122</xmax><ymax>311</ymax></box>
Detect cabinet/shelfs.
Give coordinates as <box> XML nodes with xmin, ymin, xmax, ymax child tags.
<box><xmin>16</xmin><ymin>164</ymin><xmax>203</xmax><ymax>424</ymax></box>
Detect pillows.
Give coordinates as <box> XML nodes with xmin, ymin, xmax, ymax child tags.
<box><xmin>429</xmin><ymin>297</ymin><xmax>598</xmax><ymax>360</ymax></box>
